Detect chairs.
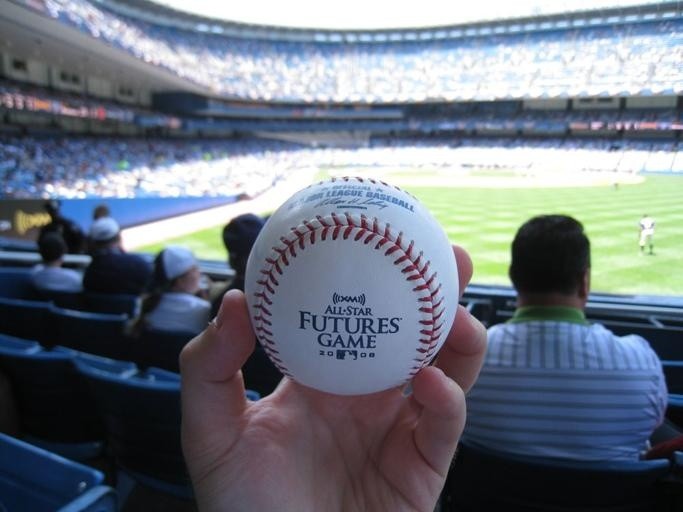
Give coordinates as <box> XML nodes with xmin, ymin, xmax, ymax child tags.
<box><xmin>0</xmin><ymin>241</ymin><xmax>683</xmax><ymax>512</ymax></box>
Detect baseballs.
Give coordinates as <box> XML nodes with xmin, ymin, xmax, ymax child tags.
<box><xmin>244</xmin><ymin>176</ymin><xmax>461</xmax><ymax>397</ymax></box>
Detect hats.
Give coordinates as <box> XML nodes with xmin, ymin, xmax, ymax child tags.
<box><xmin>90</xmin><ymin>217</ymin><xmax>120</xmax><ymax>242</ymax></box>
<box><xmin>223</xmin><ymin>214</ymin><xmax>270</xmax><ymax>252</ymax></box>
<box><xmin>149</xmin><ymin>246</ymin><xmax>200</xmax><ymax>284</ymax></box>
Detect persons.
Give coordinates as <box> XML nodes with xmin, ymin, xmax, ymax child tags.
<box><xmin>460</xmin><ymin>214</ymin><xmax>668</xmax><ymax>460</ymax></box>
<box><xmin>77</xmin><ymin>205</ymin><xmax>125</xmax><ymax>257</ymax></box>
<box><xmin>638</xmin><ymin>213</ymin><xmax>654</xmax><ymax>255</ymax></box>
<box><xmin>29</xmin><ymin>232</ymin><xmax>86</xmax><ymax>294</ymax></box>
<box><xmin>135</xmin><ymin>244</ymin><xmax>211</xmax><ymax>335</ymax></box>
<box><xmin>178</xmin><ymin>246</ymin><xmax>488</xmax><ymax>512</ymax></box>
<box><xmin>82</xmin><ymin>214</ymin><xmax>152</xmax><ymax>295</ymax></box>
<box><xmin>37</xmin><ymin>199</ymin><xmax>82</xmax><ymax>270</ymax></box>
<box><xmin>209</xmin><ymin>213</ymin><xmax>283</xmax><ymax>398</ymax></box>
